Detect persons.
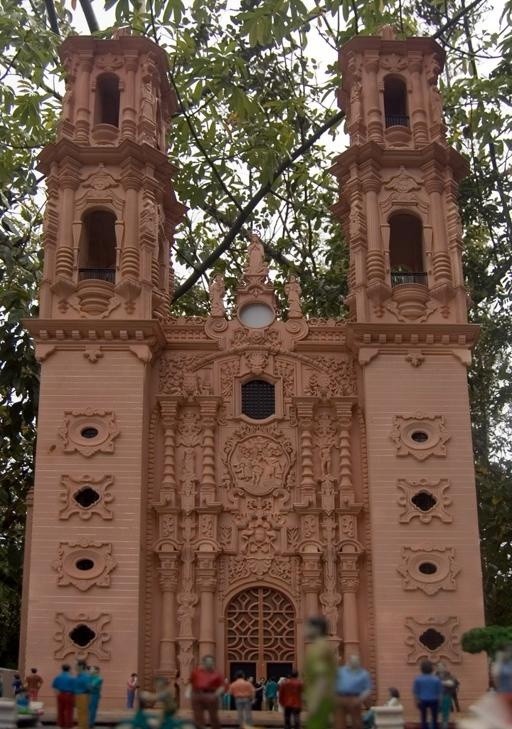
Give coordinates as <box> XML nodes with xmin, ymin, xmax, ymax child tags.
<box><xmin>246</xmin><ymin>235</ymin><xmax>265</xmax><ymax>272</ymax></box>
<box><xmin>284</xmin><ymin>273</ymin><xmax>304</xmax><ymax>313</ymax></box>
<box><xmin>208</xmin><ymin>273</ymin><xmax>226</xmax><ymax>308</ymax></box>
